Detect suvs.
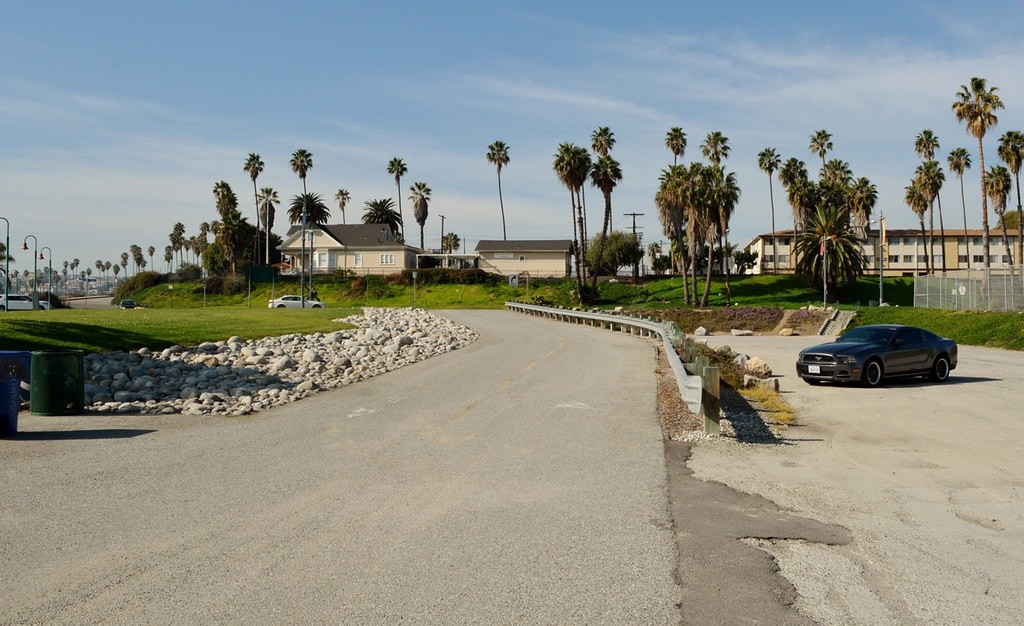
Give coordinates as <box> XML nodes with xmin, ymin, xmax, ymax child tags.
<box><xmin>0</xmin><ymin>294</ymin><xmax>52</xmax><ymax>311</ymax></box>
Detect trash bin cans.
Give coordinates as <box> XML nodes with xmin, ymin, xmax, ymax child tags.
<box><xmin>0</xmin><ymin>351</ymin><xmax>31</xmax><ymax>438</ymax></box>
<box><xmin>31</xmin><ymin>349</ymin><xmax>85</xmax><ymax>416</ymax></box>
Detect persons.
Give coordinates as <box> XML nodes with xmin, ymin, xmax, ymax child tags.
<box><xmin>309</xmin><ymin>285</ymin><xmax>317</xmax><ymax>301</ymax></box>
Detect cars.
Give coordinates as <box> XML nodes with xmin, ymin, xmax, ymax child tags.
<box><xmin>118</xmin><ymin>299</ymin><xmax>137</xmax><ymax>309</ymax></box>
<box><xmin>268</xmin><ymin>294</ymin><xmax>324</xmax><ymax>309</ymax></box>
<box><xmin>795</xmin><ymin>324</ymin><xmax>959</xmax><ymax>388</ymax></box>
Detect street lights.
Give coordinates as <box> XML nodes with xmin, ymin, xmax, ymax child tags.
<box><xmin>40</xmin><ymin>246</ymin><xmax>51</xmax><ymax>310</ymax></box>
<box><xmin>870</xmin><ymin>210</ymin><xmax>886</xmax><ymax>306</ymax></box>
<box><xmin>21</xmin><ymin>234</ymin><xmax>38</xmax><ymax>293</ymax></box>
